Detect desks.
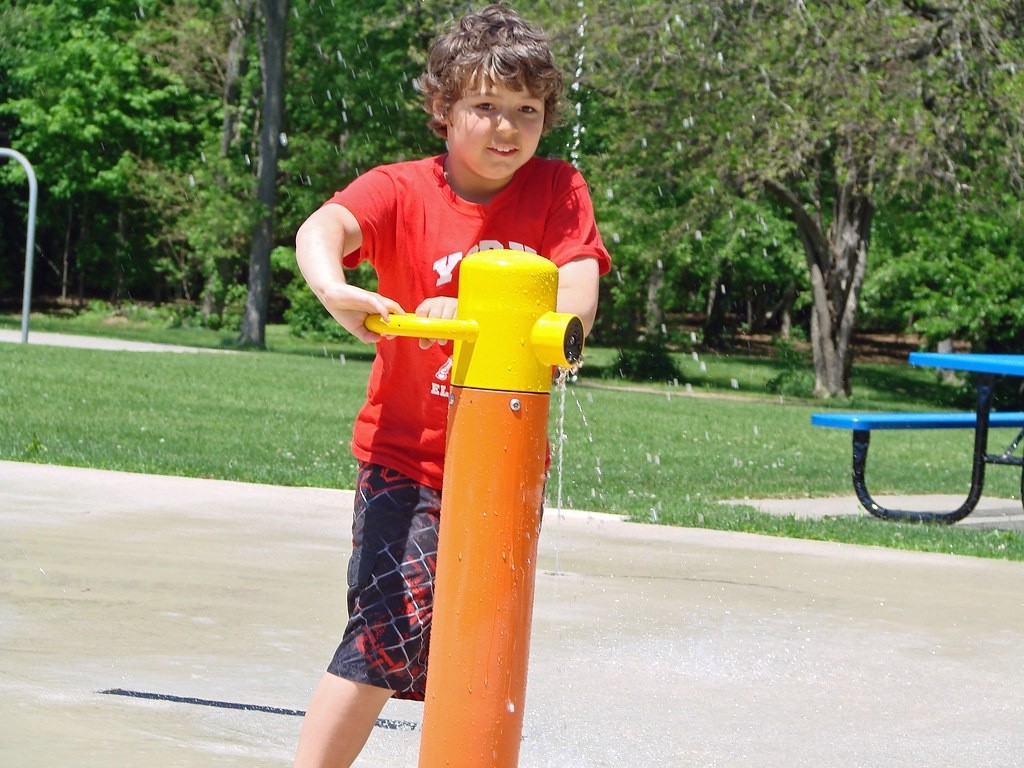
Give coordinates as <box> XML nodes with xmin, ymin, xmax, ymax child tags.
<box><xmin>908</xmin><ymin>351</ymin><xmax>1024</xmax><ymax>525</ymax></box>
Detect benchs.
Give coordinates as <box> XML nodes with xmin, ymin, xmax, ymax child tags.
<box><xmin>810</xmin><ymin>413</ymin><xmax>1024</xmax><ymax>509</ymax></box>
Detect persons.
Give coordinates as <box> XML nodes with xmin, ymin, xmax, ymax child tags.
<box><xmin>293</xmin><ymin>8</ymin><xmax>610</xmax><ymax>768</ymax></box>
